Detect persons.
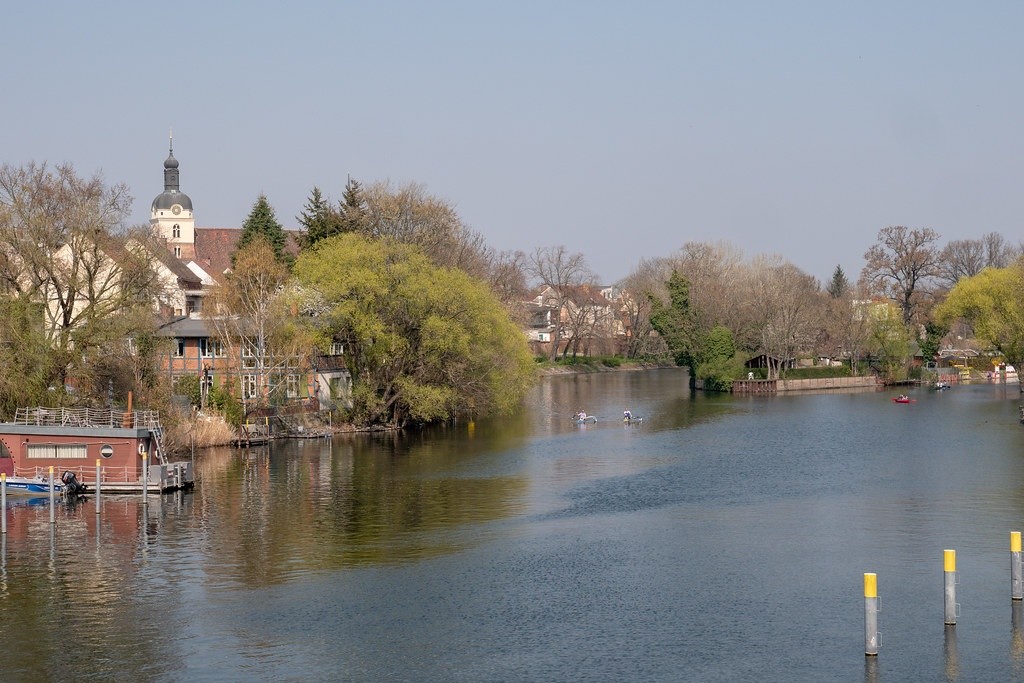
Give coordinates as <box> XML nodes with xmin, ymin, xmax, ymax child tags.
<box><xmin>936</xmin><ymin>380</ymin><xmax>946</xmax><ymax>387</ymax></box>
<box><xmin>748</xmin><ymin>371</ymin><xmax>754</xmax><ymax>380</ymax></box>
<box><xmin>624</xmin><ymin>408</ymin><xmax>632</xmax><ymax>420</ymax></box>
<box><xmin>898</xmin><ymin>393</ymin><xmax>908</xmax><ymax>400</ymax></box>
<box><xmin>576</xmin><ymin>410</ymin><xmax>586</xmax><ymax>424</ymax></box>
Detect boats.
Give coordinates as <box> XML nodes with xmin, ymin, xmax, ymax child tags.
<box><xmin>0</xmin><ymin>470</ymin><xmax>90</xmax><ymax>498</ymax></box>
<box><xmin>928</xmin><ymin>379</ymin><xmax>951</xmax><ymax>391</ymax></box>
<box><xmin>891</xmin><ymin>397</ymin><xmax>911</xmax><ymax>403</ymax></box>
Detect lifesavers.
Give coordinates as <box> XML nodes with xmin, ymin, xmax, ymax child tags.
<box><xmin>155</xmin><ymin>448</ymin><xmax>160</xmax><ymax>459</ymax></box>
<box><xmin>139</xmin><ymin>444</ymin><xmax>145</xmax><ymax>456</ymax></box>
